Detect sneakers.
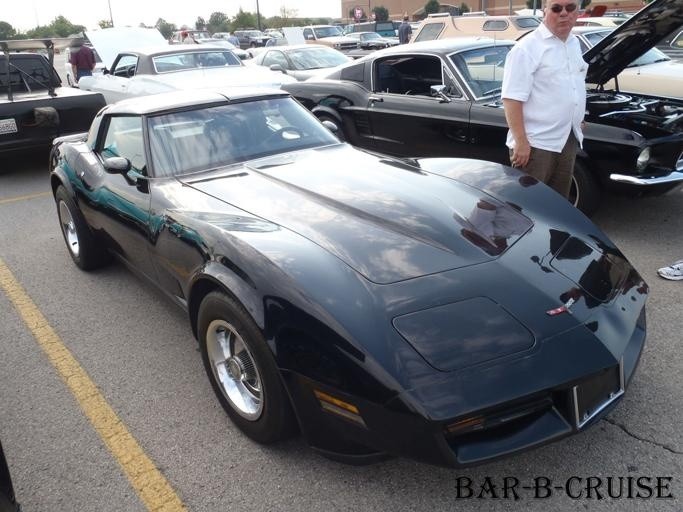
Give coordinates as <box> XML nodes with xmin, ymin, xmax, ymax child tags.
<box><xmin>657</xmin><ymin>262</ymin><xmax>683</xmax><ymax>282</ymax></box>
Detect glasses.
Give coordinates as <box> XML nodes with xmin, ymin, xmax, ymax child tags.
<box><xmin>546</xmin><ymin>3</ymin><xmax>579</xmax><ymax>14</ymax></box>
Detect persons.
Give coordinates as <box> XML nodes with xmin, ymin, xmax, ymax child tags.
<box><xmin>397</xmin><ymin>15</ymin><xmax>411</xmax><ymax>44</ymax></box>
<box><xmin>498</xmin><ymin>1</ymin><xmax>590</xmax><ymax>204</ymax></box>
<box><xmin>68</xmin><ymin>36</ymin><xmax>97</xmax><ymax>85</ymax></box>
<box><xmin>227</xmin><ymin>30</ymin><xmax>240</xmax><ymax>49</ymax></box>
